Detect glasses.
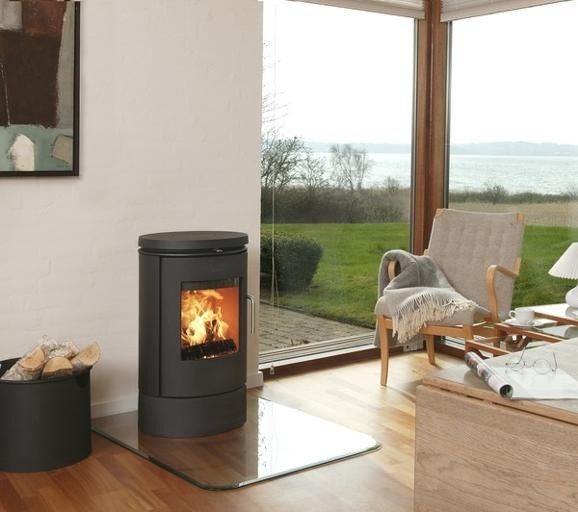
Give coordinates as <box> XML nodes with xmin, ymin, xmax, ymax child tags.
<box><xmin>506</xmin><ymin>346</ymin><xmax>558</xmax><ymax>375</ymax></box>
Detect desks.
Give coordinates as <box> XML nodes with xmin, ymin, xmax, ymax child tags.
<box><xmin>465</xmin><ymin>300</ymin><xmax>578</xmax><ymax>354</ymax></box>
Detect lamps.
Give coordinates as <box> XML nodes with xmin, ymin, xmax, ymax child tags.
<box><xmin>547</xmin><ymin>242</ymin><xmax>578</xmax><ymax>309</ymax></box>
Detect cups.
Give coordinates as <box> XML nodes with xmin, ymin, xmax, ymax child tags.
<box><xmin>511</xmin><ymin>310</ymin><xmax>534</xmax><ymax>323</ymax></box>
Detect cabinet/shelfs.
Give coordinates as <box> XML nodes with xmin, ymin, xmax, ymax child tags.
<box><xmin>415</xmin><ymin>364</ymin><xmax>578</xmax><ymax>512</ymax></box>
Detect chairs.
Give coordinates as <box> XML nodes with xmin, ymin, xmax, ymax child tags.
<box><xmin>374</xmin><ymin>208</ymin><xmax>524</xmax><ymax>387</ymax></box>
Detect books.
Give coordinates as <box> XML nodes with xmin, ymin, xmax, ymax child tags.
<box><xmin>464</xmin><ymin>337</ymin><xmax>578</xmax><ymax>401</ymax></box>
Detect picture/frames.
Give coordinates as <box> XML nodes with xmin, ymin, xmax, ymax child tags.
<box><xmin>0</xmin><ymin>0</ymin><xmax>80</xmax><ymax>177</ymax></box>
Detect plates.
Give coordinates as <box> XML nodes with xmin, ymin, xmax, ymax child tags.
<box><xmin>506</xmin><ymin>319</ymin><xmax>544</xmax><ymax>330</ymax></box>
